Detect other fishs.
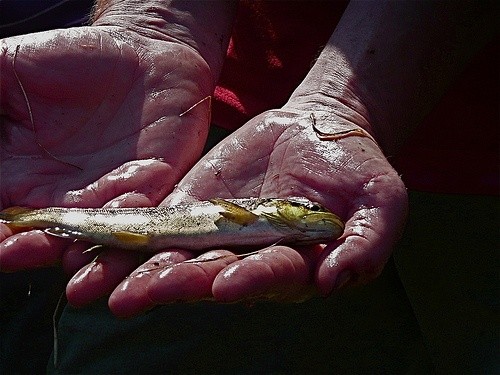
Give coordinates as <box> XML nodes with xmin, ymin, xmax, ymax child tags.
<box><xmin>1</xmin><ymin>195</ymin><xmax>346</xmax><ymax>253</ymax></box>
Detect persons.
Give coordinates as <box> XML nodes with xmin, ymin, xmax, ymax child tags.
<box><xmin>0</xmin><ymin>1</ymin><xmax>500</xmax><ymax>375</ymax></box>
